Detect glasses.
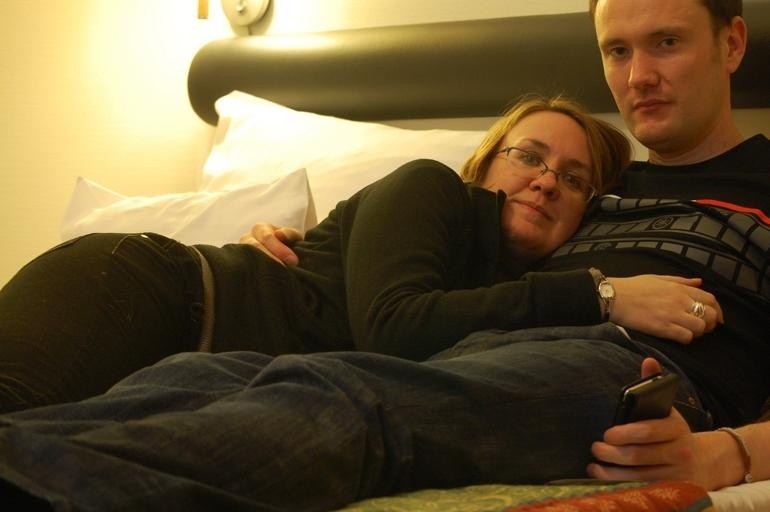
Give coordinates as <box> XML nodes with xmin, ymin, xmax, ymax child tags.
<box><xmin>494</xmin><ymin>146</ymin><xmax>598</xmax><ymax>204</ymax></box>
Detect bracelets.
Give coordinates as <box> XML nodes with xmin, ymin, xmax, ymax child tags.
<box><xmin>715</xmin><ymin>425</ymin><xmax>752</xmax><ymax>486</ymax></box>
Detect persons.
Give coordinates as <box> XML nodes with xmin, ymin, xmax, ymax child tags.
<box><xmin>0</xmin><ymin>93</ymin><xmax>725</xmax><ymax>424</ymax></box>
<box><xmin>2</xmin><ymin>0</ymin><xmax>770</xmax><ymax>512</ymax></box>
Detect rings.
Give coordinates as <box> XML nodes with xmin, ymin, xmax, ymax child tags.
<box><xmin>690</xmin><ymin>301</ymin><xmax>705</xmax><ymax>319</ymax></box>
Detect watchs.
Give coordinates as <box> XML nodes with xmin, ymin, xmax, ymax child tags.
<box><xmin>598</xmin><ymin>278</ymin><xmax>616</xmax><ymax>322</ymax></box>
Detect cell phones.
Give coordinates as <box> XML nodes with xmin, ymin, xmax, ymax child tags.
<box><xmin>601</xmin><ymin>370</ymin><xmax>679</xmax><ymax>469</ymax></box>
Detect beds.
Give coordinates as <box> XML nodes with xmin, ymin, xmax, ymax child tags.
<box><xmin>185</xmin><ymin>0</ymin><xmax>770</xmax><ymax>512</ymax></box>
